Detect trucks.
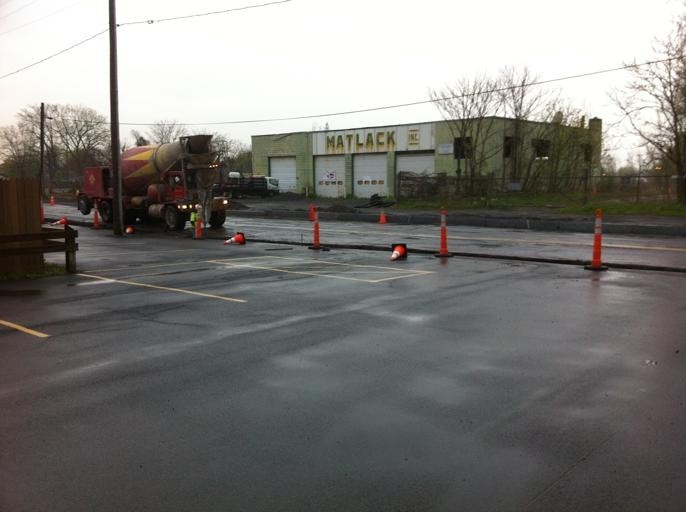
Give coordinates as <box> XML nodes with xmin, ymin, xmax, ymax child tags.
<box><xmin>212</xmin><ymin>172</ymin><xmax>279</xmax><ymax>198</ymax></box>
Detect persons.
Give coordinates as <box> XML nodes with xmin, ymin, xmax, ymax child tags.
<box><xmin>188</xmin><ymin>204</ymin><xmax>204</xmax><ymax>240</ymax></box>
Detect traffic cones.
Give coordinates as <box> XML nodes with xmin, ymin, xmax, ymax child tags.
<box><xmin>50</xmin><ymin>195</ymin><xmax>55</xmax><ymax>205</ymax></box>
<box><xmin>389</xmin><ymin>243</ymin><xmax>407</xmax><ymax>260</ymax></box>
<box><xmin>51</xmin><ymin>217</ymin><xmax>67</xmax><ymax>226</ymax></box>
<box><xmin>40</xmin><ymin>199</ymin><xmax>44</xmax><ymax>223</ymax></box>
<box><xmin>222</xmin><ymin>232</ymin><xmax>246</xmax><ymax>246</ymax></box>
<box><xmin>91</xmin><ymin>210</ymin><xmax>99</xmax><ymax>228</ymax></box>
<box><xmin>379</xmin><ymin>205</ymin><xmax>386</xmax><ymax>224</ymax></box>
<box><xmin>124</xmin><ymin>225</ymin><xmax>134</xmax><ymax>234</ymax></box>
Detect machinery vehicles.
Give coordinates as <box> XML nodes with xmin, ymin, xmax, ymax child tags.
<box><xmin>76</xmin><ymin>135</ymin><xmax>228</xmax><ymax>230</ymax></box>
